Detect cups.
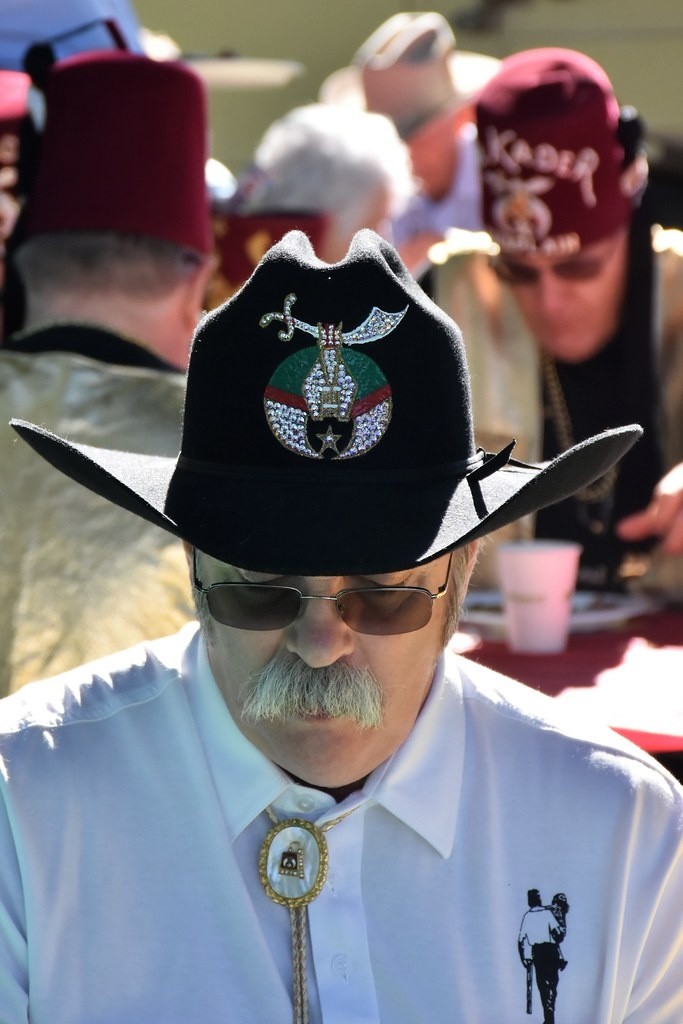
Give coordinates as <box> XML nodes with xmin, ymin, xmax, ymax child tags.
<box><xmin>499</xmin><ymin>539</ymin><xmax>583</xmax><ymax>653</ymax></box>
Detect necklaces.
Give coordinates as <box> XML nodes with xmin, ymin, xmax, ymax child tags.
<box><xmin>254</xmin><ymin>803</ymin><xmax>359</xmax><ymax>1024</ymax></box>
<box><xmin>546</xmin><ymin>356</ymin><xmax>617</xmax><ymax>503</ymax></box>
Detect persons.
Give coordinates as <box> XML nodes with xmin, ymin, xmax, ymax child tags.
<box><xmin>0</xmin><ymin>5</ymin><xmax>683</xmax><ymax>701</ymax></box>
<box><xmin>1</xmin><ymin>230</ymin><xmax>683</xmax><ymax>1024</ymax></box>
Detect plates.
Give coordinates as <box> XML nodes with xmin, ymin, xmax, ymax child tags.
<box><xmin>459</xmin><ymin>590</ymin><xmax>655</xmax><ymax>642</ymax></box>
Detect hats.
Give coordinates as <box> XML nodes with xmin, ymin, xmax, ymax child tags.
<box><xmin>10</xmin><ymin>50</ymin><xmax>214</xmax><ymax>266</ymax></box>
<box><xmin>474</xmin><ymin>45</ymin><xmax>649</xmax><ymax>253</ymax></box>
<box><xmin>10</xmin><ymin>227</ymin><xmax>643</xmax><ymax>576</ymax></box>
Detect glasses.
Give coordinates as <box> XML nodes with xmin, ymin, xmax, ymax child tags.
<box><xmin>487</xmin><ymin>226</ymin><xmax>626</xmax><ymax>288</ymax></box>
<box><xmin>191</xmin><ymin>545</ymin><xmax>453</xmax><ymax>635</ymax></box>
